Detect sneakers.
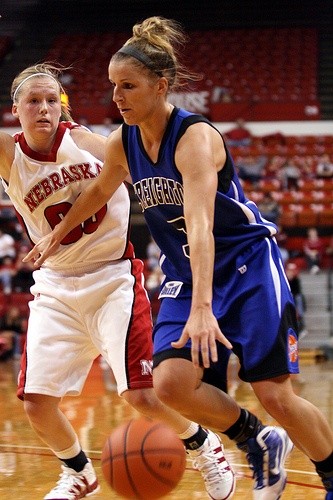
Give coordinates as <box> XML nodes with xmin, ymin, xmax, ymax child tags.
<box><xmin>184</xmin><ymin>425</ymin><xmax>235</xmax><ymax>500</ymax></box>
<box><xmin>44</xmin><ymin>456</ymin><xmax>100</xmax><ymax>500</ymax></box>
<box><xmin>236</xmin><ymin>413</ymin><xmax>295</xmax><ymax>500</ymax></box>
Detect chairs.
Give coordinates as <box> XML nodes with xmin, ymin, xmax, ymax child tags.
<box><xmin>0</xmin><ymin>21</ymin><xmax>333</xmax><ymax>352</ymax></box>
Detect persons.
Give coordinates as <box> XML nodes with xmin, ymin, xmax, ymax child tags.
<box><xmin>0</xmin><ymin>64</ymin><xmax>237</xmax><ymax>500</ymax></box>
<box><xmin>0</xmin><ymin>77</ymin><xmax>333</xmax><ymax>361</ymax></box>
<box><xmin>22</xmin><ymin>17</ymin><xmax>333</xmax><ymax>500</ymax></box>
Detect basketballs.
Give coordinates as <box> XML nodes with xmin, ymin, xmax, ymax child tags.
<box><xmin>101</xmin><ymin>419</ymin><xmax>188</xmax><ymax>500</ymax></box>
